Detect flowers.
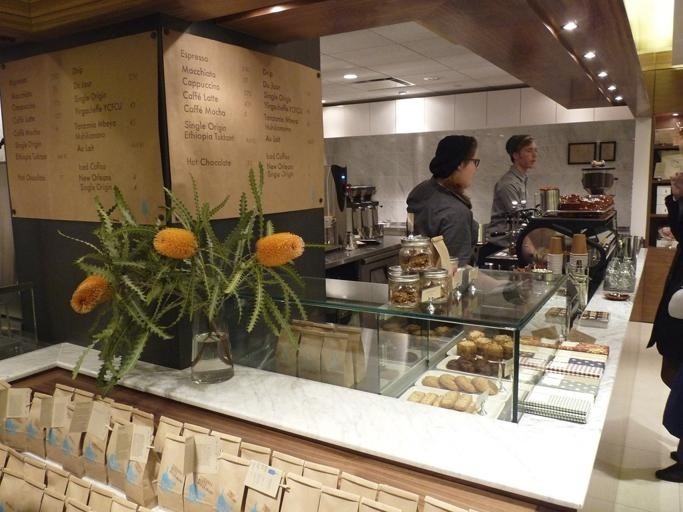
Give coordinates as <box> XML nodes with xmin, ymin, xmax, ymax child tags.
<box><xmin>56</xmin><ymin>162</ymin><xmax>347</xmax><ymax>402</ymax></box>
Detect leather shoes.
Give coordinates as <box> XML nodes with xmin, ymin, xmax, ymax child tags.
<box><xmin>655</xmin><ymin>451</ymin><xmax>683</xmax><ymax>483</ymax></box>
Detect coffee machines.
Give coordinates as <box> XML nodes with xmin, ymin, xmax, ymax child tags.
<box><xmin>345</xmin><ymin>184</ymin><xmax>384</xmax><ymax>241</ymax></box>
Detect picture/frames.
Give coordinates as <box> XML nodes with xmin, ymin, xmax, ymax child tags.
<box><xmin>568</xmin><ymin>142</ymin><xmax>596</xmax><ymax>164</ymax></box>
<box><xmin>599</xmin><ymin>141</ymin><xmax>616</xmax><ymax>161</ymax></box>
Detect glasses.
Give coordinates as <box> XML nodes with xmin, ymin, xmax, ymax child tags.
<box><xmin>471</xmin><ymin>158</ymin><xmax>480</xmax><ymax>167</ymax></box>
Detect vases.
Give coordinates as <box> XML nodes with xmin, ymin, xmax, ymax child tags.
<box><xmin>192</xmin><ymin>319</ymin><xmax>234</xmax><ymax>385</ymax></box>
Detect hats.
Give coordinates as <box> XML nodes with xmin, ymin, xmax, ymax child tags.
<box><xmin>505</xmin><ymin>134</ymin><xmax>531</xmax><ymax>156</ymax></box>
<box><xmin>429</xmin><ymin>135</ymin><xmax>468</xmax><ymax>176</ymax></box>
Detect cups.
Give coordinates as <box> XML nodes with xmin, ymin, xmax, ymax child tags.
<box><xmin>603</xmin><ymin>256</ymin><xmax>636</xmax><ymax>292</ymax></box>
<box><xmin>547</xmin><ymin>235</ymin><xmax>588</xmax><ymax>274</ymax></box>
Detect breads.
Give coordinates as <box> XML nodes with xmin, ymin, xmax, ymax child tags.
<box><xmin>359</xmin><ymin>312</ymin><xmax>452</xmax><ymax>383</ymax></box>
<box><xmin>409</xmin><ymin>330</ymin><xmax>514</xmax><ymax>413</ymax></box>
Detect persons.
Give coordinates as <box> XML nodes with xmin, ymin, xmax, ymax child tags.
<box><xmin>488</xmin><ymin>133</ymin><xmax>544</xmax><ymax>264</ymax></box>
<box><xmin>655</xmin><ymin>368</ymin><xmax>682</xmax><ymax>485</ymax></box>
<box><xmin>643</xmin><ymin>167</ymin><xmax>683</xmax><ymax>390</ymax></box>
<box><xmin>404</xmin><ymin>134</ymin><xmax>513</xmax><ymax>292</ymax></box>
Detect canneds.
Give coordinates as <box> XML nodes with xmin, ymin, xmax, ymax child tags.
<box><xmin>387</xmin><ymin>236</ymin><xmax>453</xmax><ymax>308</ymax></box>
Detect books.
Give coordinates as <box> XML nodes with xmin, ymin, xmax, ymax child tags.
<box><xmin>579</xmin><ymin>310</ymin><xmax>610</xmax><ymax>329</ymax></box>
<box><xmin>545</xmin><ymin>307</ymin><xmax>568</xmax><ymax>324</ymax></box>
<box><xmin>516</xmin><ymin>333</ymin><xmax>559</xmax><ymax>412</ymax></box>
<box><xmin>523</xmin><ymin>340</ymin><xmax>611</xmax><ymax>423</ymax></box>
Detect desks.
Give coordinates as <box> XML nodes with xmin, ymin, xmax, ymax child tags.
<box><xmin>325</xmin><ymin>236</ymin><xmax>407</xmax><ymax>283</ymax></box>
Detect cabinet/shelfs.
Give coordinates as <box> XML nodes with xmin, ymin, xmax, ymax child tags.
<box><xmin>221</xmin><ymin>296</ymin><xmax>428</xmax><ymax>398</ymax></box>
<box><xmin>642</xmin><ymin>114</ymin><xmax>683</xmax><ymax>325</ymax></box>
<box><xmin>378</xmin><ymin>269</ymin><xmax>571</xmax><ymax>423</ymax></box>
